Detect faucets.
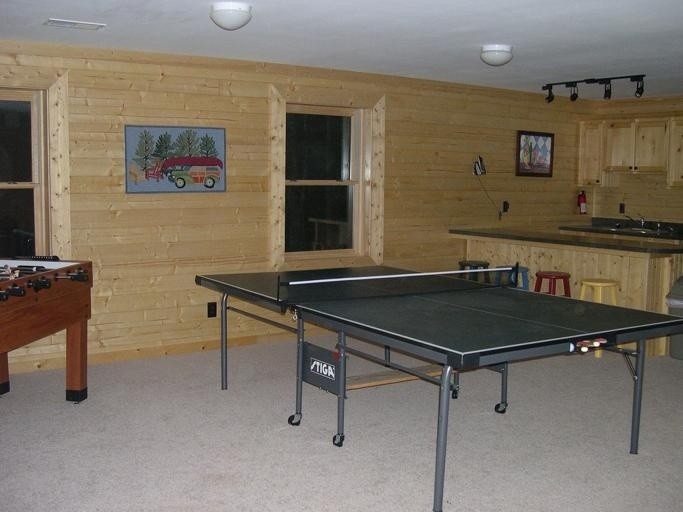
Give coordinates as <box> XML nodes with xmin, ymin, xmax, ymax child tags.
<box><xmin>624</xmin><ymin>213</ymin><xmax>644</xmax><ymax>227</ymax></box>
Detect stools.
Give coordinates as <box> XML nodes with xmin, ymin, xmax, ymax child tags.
<box><xmin>457</xmin><ymin>257</ymin><xmax>619</xmax><ymax>357</ymax></box>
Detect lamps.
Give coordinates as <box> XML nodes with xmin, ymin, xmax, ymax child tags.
<box><xmin>209</xmin><ymin>3</ymin><xmax>253</xmax><ymax>31</ymax></box>
<box><xmin>482</xmin><ymin>44</ymin><xmax>513</xmax><ymax>67</ymax></box>
<box><xmin>541</xmin><ymin>76</ymin><xmax>646</xmax><ymax>103</ymax></box>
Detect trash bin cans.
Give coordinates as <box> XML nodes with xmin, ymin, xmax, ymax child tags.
<box><xmin>665</xmin><ymin>276</ymin><xmax>683</xmax><ymax>360</ymax></box>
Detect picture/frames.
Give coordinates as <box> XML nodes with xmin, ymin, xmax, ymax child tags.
<box><xmin>124</xmin><ymin>124</ymin><xmax>227</xmax><ymax>194</ymax></box>
<box><xmin>516</xmin><ymin>131</ymin><xmax>554</xmax><ymax>177</ymax></box>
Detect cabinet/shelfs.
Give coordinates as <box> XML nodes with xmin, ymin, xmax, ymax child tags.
<box><xmin>576</xmin><ymin>115</ymin><xmax>617</xmax><ymax>188</ymax></box>
<box><xmin>604</xmin><ymin>113</ymin><xmax>668</xmax><ymax>174</ymax></box>
<box><xmin>666</xmin><ymin>111</ymin><xmax>683</xmax><ymax>188</ymax></box>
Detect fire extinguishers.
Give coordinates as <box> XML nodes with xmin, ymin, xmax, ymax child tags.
<box><xmin>577</xmin><ymin>190</ymin><xmax>587</xmax><ymax>214</ymax></box>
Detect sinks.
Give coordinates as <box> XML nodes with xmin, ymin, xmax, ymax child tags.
<box><xmin>608</xmin><ymin>228</ymin><xmax>655</xmax><ymax>233</ymax></box>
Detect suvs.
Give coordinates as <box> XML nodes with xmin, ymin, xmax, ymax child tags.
<box><xmin>168</xmin><ymin>166</ymin><xmax>220</xmax><ymax>188</ymax></box>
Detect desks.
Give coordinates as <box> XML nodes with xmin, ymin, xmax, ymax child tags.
<box><xmin>195</xmin><ymin>265</ymin><xmax>683</xmax><ymax>512</ymax></box>
